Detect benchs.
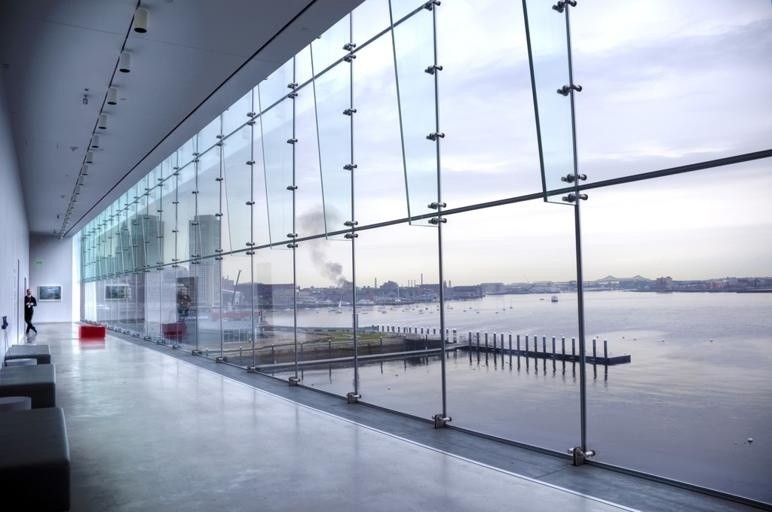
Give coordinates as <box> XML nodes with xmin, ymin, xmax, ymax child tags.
<box><xmin>0</xmin><ymin>344</ymin><xmax>73</xmax><ymax>511</ymax></box>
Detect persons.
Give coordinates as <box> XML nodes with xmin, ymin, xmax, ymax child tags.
<box><xmin>24</xmin><ymin>288</ymin><xmax>37</xmax><ymax>337</ymax></box>
<box><xmin>175</xmin><ymin>284</ymin><xmax>193</xmax><ymax>342</ymax></box>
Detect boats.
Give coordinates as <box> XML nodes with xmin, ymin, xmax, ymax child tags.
<box><xmin>551</xmin><ymin>295</ymin><xmax>558</xmax><ymax>302</ymax></box>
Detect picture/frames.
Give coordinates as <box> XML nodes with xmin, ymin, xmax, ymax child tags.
<box><xmin>37</xmin><ymin>285</ymin><xmax>63</xmax><ymax>302</ymax></box>
